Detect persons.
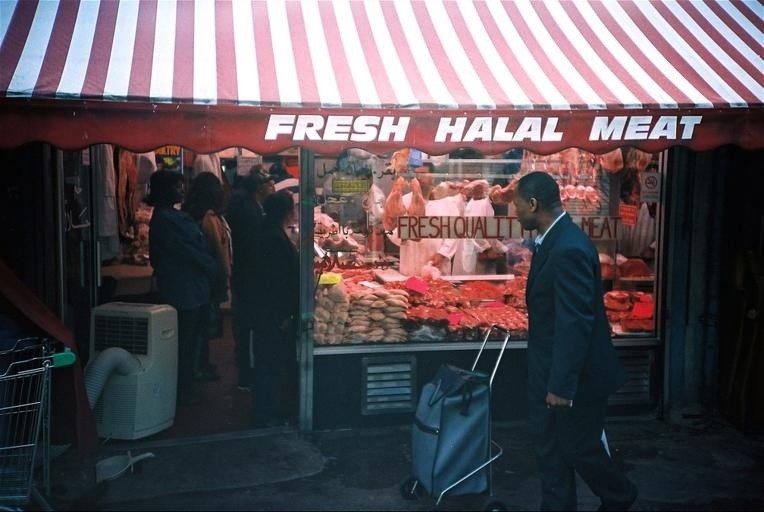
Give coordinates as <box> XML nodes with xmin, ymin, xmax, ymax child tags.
<box><xmin>455</xmin><ymin>170</ymin><xmax>504</xmax><ymax>277</ymax></box>
<box><xmin>512</xmin><ymin>170</ymin><xmax>639</xmax><ymax>512</ymax></box>
<box><xmin>141</xmin><ymin>161</ymin><xmax>300</xmax><ymax>428</ymax></box>
<box><xmin>384</xmin><ymin>163</ymin><xmax>464</xmax><ymax>276</ymax></box>
<box><xmin>356</xmin><ymin>169</ymin><xmax>387</xmax><ymax>252</ymax></box>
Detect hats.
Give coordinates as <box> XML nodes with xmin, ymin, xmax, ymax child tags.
<box><xmin>251</xmin><ymin>170</ymin><xmax>275</xmax><ymax>185</ymax></box>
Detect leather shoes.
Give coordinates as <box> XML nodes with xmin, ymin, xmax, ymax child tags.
<box><xmin>597</xmin><ymin>485</ymin><xmax>637</xmax><ymax>511</ymax></box>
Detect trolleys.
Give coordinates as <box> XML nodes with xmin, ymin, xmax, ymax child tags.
<box><xmin>399</xmin><ymin>324</ymin><xmax>511</xmax><ymax>512</ymax></box>
<box><xmin>0</xmin><ymin>336</ymin><xmax>77</xmax><ymax>512</ymax></box>
